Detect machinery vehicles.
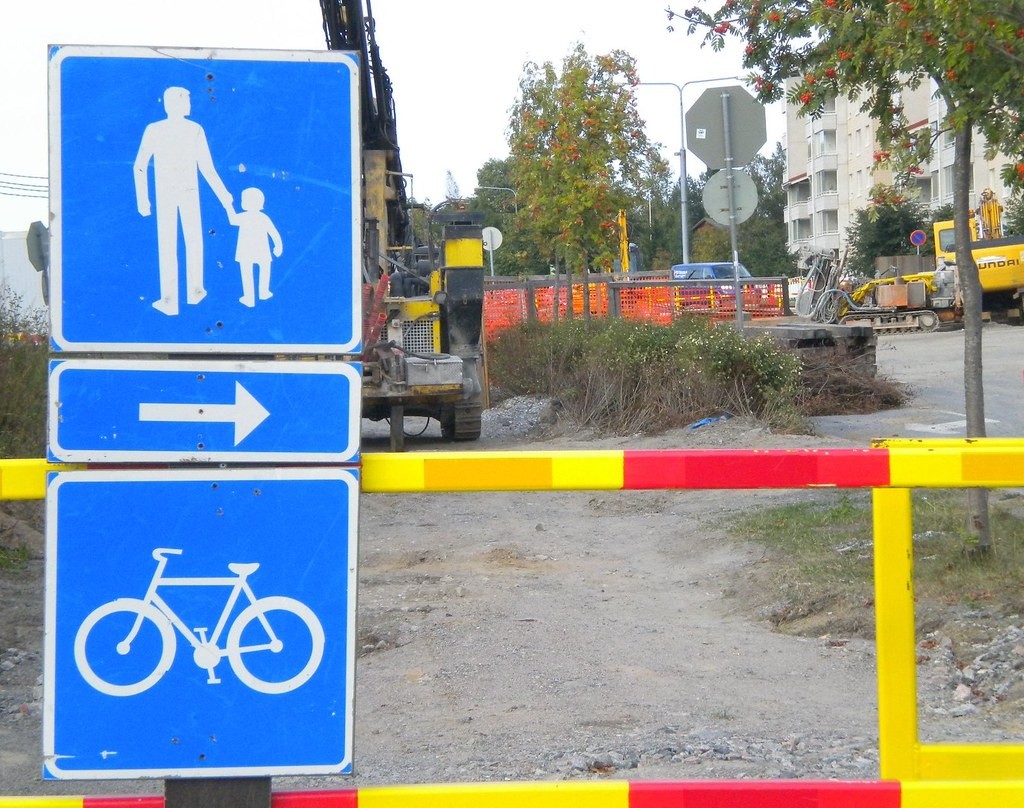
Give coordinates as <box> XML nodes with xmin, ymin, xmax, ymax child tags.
<box><xmin>834</xmin><ymin>217</ymin><xmax>1024</xmax><ymax>333</ymax></box>
<box><xmin>276</xmin><ymin>0</ymin><xmax>494</xmax><ymax>453</ymax></box>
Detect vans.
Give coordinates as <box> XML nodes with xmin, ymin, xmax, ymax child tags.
<box><xmin>671</xmin><ymin>256</ymin><xmax>761</xmax><ymax>310</ymax></box>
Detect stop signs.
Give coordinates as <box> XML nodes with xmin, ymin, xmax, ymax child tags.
<box><xmin>911</xmin><ymin>230</ymin><xmax>927</xmax><ymax>246</ymax></box>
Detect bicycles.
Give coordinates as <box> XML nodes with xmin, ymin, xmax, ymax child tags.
<box><xmin>73</xmin><ymin>546</ymin><xmax>326</xmax><ymax>698</ymax></box>
